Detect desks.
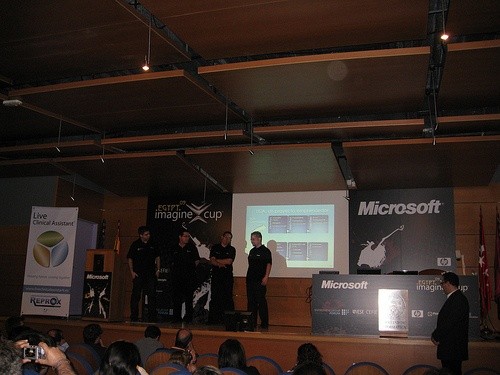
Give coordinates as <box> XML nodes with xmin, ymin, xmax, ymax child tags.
<box><xmin>312</xmin><ymin>274</ymin><xmax>482</xmax><ymax>340</ymax></box>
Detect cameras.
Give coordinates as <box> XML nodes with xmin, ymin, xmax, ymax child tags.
<box><xmin>23</xmin><ymin>345</ymin><xmax>42</xmax><ymax>360</ymax></box>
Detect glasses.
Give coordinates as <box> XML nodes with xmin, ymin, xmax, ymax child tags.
<box><xmin>440</xmin><ymin>282</ymin><xmax>446</xmax><ymax>285</ymax></box>
<box><xmin>184</xmin><ymin>235</ymin><xmax>190</xmax><ymax>238</ymax></box>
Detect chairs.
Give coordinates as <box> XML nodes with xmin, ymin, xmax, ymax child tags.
<box><xmin>292</xmin><ymin>362</ymin><xmax>335</xmax><ymax>375</ymax></box>
<box><xmin>344</xmin><ymin>362</ymin><xmax>390</xmax><ymax>375</ymax></box>
<box><xmin>403</xmin><ymin>364</ymin><xmax>441</xmax><ymax>375</ymax></box>
<box><xmin>245</xmin><ymin>356</ymin><xmax>284</xmax><ymax>375</ymax></box>
<box><xmin>14</xmin><ymin>334</ymin><xmax>247</xmax><ymax>375</ymax></box>
<box><xmin>465</xmin><ymin>367</ymin><xmax>500</xmax><ymax>375</ymax></box>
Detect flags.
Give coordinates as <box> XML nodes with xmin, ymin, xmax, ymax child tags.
<box><xmin>112</xmin><ymin>220</ymin><xmax>121</xmax><ymax>256</ymax></box>
<box><xmin>494</xmin><ymin>205</ymin><xmax>500</xmax><ymax>307</ymax></box>
<box><xmin>478</xmin><ymin>206</ymin><xmax>492</xmax><ymax>315</ymax></box>
<box><xmin>97</xmin><ymin>218</ymin><xmax>105</xmax><ymax>248</ymax></box>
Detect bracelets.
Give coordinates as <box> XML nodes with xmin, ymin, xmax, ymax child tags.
<box><xmin>157</xmin><ymin>270</ymin><xmax>160</xmax><ymax>272</ymax></box>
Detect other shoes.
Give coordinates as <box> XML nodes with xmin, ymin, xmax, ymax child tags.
<box><xmin>258</xmin><ymin>323</ymin><xmax>269</xmax><ymax>329</ymax></box>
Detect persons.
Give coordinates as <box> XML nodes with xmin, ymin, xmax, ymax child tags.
<box><xmin>293</xmin><ymin>343</ymin><xmax>326</xmax><ymax>375</ymax></box>
<box><xmin>126</xmin><ymin>226</ymin><xmax>161</xmax><ymax>323</ymax></box>
<box><xmin>431</xmin><ymin>272</ymin><xmax>469</xmax><ymax>375</ymax></box>
<box><xmin>166</xmin><ymin>230</ymin><xmax>200</xmax><ymax>326</ymax></box>
<box><xmin>423</xmin><ymin>367</ymin><xmax>456</xmax><ymax>375</ymax></box>
<box><xmin>246</xmin><ymin>231</ymin><xmax>272</xmax><ymax>329</ymax></box>
<box><xmin>204</xmin><ymin>232</ymin><xmax>236</xmax><ymax>325</ymax></box>
<box><xmin>0</xmin><ymin>317</ymin><xmax>260</xmax><ymax>375</ymax></box>
